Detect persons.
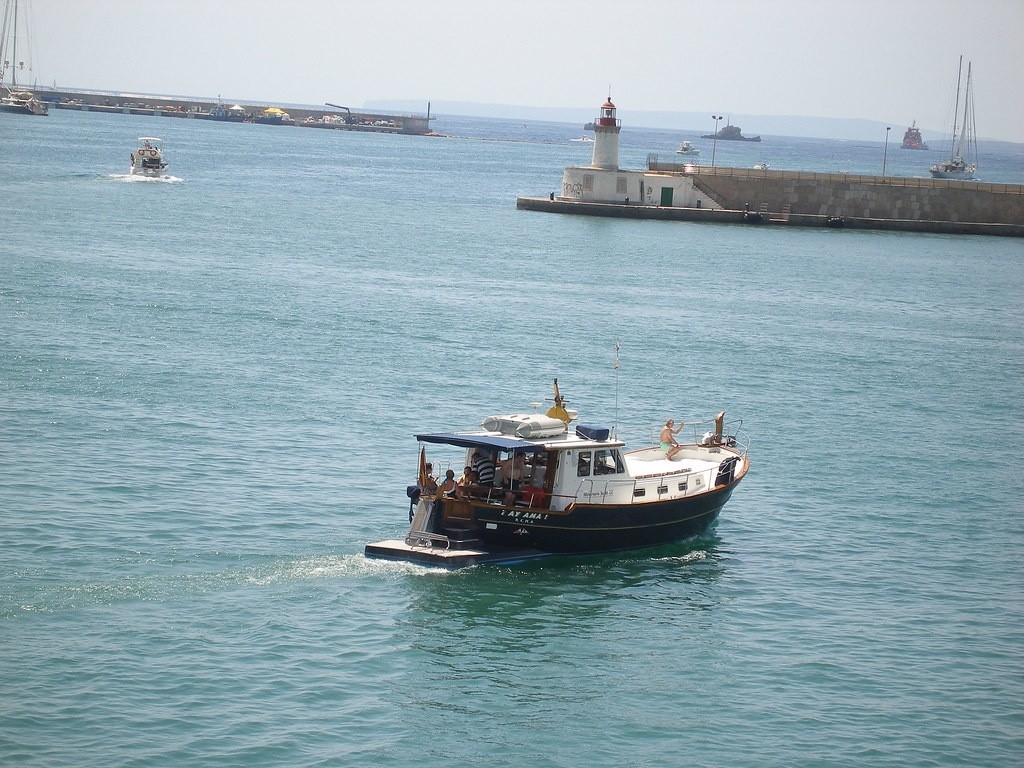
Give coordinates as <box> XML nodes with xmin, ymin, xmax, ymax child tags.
<box><xmin>145</xmin><ymin>140</ymin><xmax>150</xmax><ymax>149</ymax></box>
<box><xmin>660</xmin><ymin>419</ymin><xmax>684</xmax><ymax>460</ymax></box>
<box><xmin>499</xmin><ymin>453</ymin><xmax>527</xmax><ymax>506</ymax></box>
<box><xmin>457</xmin><ymin>467</ymin><xmax>474</xmax><ymax>497</ymax></box>
<box><xmin>471</xmin><ymin>453</ymin><xmax>494</xmax><ymax>487</ymax></box>
<box><xmin>442</xmin><ymin>470</ymin><xmax>459</xmax><ymax>499</ymax></box>
<box><xmin>426</xmin><ymin>463</ymin><xmax>436</xmax><ymax>489</ymax></box>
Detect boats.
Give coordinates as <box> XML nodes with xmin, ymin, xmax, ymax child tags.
<box><xmin>205</xmin><ymin>95</ymin><xmax>245</xmax><ymax>121</ymax></box>
<box><xmin>900</xmin><ymin>122</ymin><xmax>928</xmax><ymax>150</ymax></box>
<box><xmin>364</xmin><ymin>338</ymin><xmax>751</xmax><ymax>573</ymax></box>
<box><xmin>253</xmin><ymin>108</ymin><xmax>285</xmax><ymax>124</ymax></box>
<box><xmin>130</xmin><ymin>137</ymin><xmax>168</xmax><ymax>178</ymax></box>
<box><xmin>676</xmin><ymin>141</ymin><xmax>700</xmax><ymax>154</ymax></box>
<box><xmin>585</xmin><ymin>122</ymin><xmax>596</xmax><ymax>130</ymax></box>
<box><xmin>754</xmin><ymin>161</ymin><xmax>771</xmax><ymax>169</ymax></box>
<box><xmin>702</xmin><ymin>118</ymin><xmax>762</xmax><ymax>142</ymax></box>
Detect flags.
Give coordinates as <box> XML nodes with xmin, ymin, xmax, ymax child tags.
<box><xmin>419</xmin><ymin>449</ymin><xmax>425</xmax><ymax>487</ymax></box>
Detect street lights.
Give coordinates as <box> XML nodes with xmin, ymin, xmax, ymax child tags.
<box><xmin>712</xmin><ymin>115</ymin><xmax>722</xmax><ymax>166</ymax></box>
<box><xmin>883</xmin><ymin>126</ymin><xmax>891</xmax><ymax>177</ymax></box>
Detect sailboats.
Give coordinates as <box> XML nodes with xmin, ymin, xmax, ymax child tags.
<box><xmin>929</xmin><ymin>53</ymin><xmax>980</xmax><ymax>179</ymax></box>
<box><xmin>0</xmin><ymin>0</ymin><xmax>49</xmax><ymax>115</ymax></box>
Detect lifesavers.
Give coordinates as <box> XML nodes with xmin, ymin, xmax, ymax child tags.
<box><xmin>150</xmin><ymin>150</ymin><xmax>156</xmax><ymax>156</ymax></box>
<box><xmin>138</xmin><ymin>150</ymin><xmax>146</xmax><ymax>155</ymax></box>
